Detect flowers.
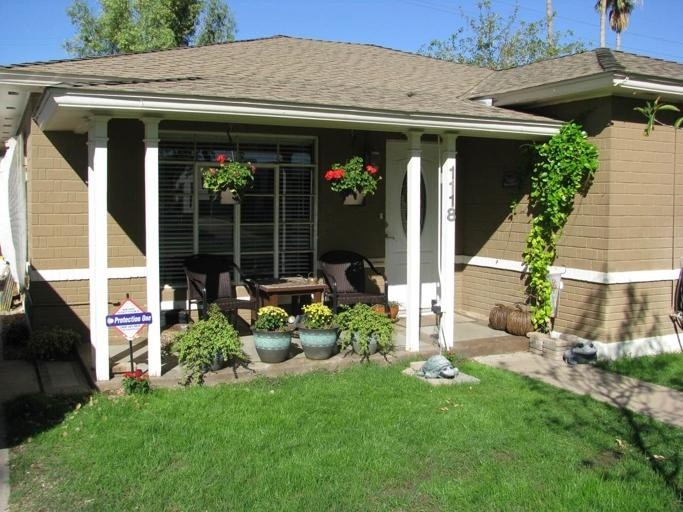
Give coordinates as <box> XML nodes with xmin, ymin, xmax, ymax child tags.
<box><xmin>199</xmin><ymin>154</ymin><xmax>256</xmax><ymax>201</ymax></box>
<box><xmin>324</xmin><ymin>154</ymin><xmax>383</xmax><ymax>197</ymax></box>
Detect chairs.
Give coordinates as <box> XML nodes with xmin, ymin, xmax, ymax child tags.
<box><xmin>182</xmin><ymin>249</ymin><xmax>391</xmax><ymax>335</ymax></box>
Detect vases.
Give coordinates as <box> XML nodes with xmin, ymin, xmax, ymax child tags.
<box><xmin>337</xmin><ymin>185</ymin><xmax>368</xmax><ymax>206</ymax></box>
<box><xmin>219</xmin><ymin>187</ymin><xmax>242</xmax><ymax>204</ymax></box>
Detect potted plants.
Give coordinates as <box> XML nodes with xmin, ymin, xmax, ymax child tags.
<box><xmin>169</xmin><ymin>302</ymin><xmax>393</xmax><ymax>386</ymax></box>
